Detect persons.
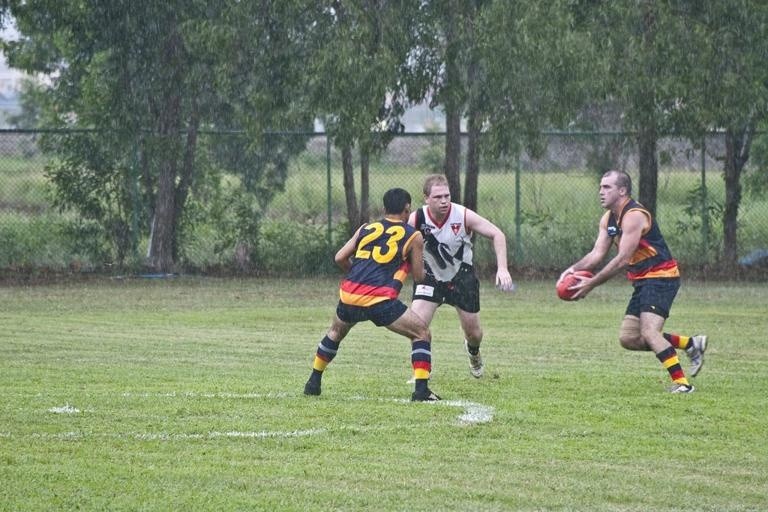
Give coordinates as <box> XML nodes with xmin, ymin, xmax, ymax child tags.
<box><xmin>557</xmin><ymin>170</ymin><xmax>708</xmax><ymax>392</ymax></box>
<box><xmin>407</xmin><ymin>176</ymin><xmax>513</xmax><ymax>383</ymax></box>
<box><xmin>304</xmin><ymin>189</ymin><xmax>441</xmax><ymax>401</ymax></box>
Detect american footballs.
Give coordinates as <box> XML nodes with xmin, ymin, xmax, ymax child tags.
<box><xmin>557</xmin><ymin>270</ymin><xmax>593</xmax><ymax>300</ymax></box>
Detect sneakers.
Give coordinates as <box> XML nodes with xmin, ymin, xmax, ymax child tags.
<box><xmin>466</xmin><ymin>342</ymin><xmax>485</xmax><ymax>378</ymax></box>
<box><xmin>304</xmin><ymin>380</ymin><xmax>321</xmax><ymax>396</ymax></box>
<box><xmin>412</xmin><ymin>390</ymin><xmax>441</xmax><ymax>401</ymax></box>
<box><xmin>686</xmin><ymin>335</ymin><xmax>708</xmax><ymax>377</ymax></box>
<box><xmin>668</xmin><ymin>383</ymin><xmax>695</xmax><ymax>394</ymax></box>
